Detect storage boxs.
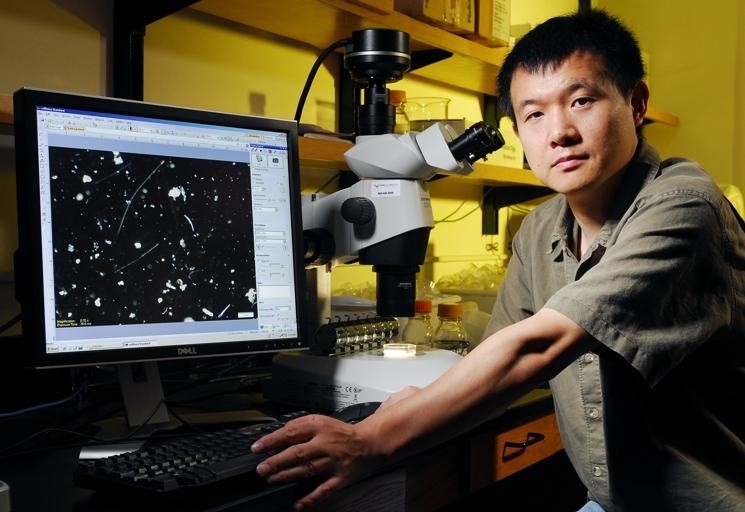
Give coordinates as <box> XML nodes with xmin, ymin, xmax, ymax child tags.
<box><xmin>395</xmin><ymin>1</ymin><xmax>511</xmax><ymax>50</ymax></box>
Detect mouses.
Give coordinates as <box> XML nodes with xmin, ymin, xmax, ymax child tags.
<box><xmin>329</xmin><ymin>401</ymin><xmax>382</xmax><ymax>424</ymax></box>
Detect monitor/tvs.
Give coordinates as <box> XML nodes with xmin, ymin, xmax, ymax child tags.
<box><xmin>14</xmin><ymin>87</ymin><xmax>312</xmax><ymax>461</ymax></box>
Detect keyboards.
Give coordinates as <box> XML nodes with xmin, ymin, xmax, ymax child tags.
<box><xmin>72</xmin><ymin>409</ymin><xmax>312</xmax><ymax>496</ymax></box>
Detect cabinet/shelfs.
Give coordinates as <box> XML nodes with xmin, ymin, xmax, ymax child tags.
<box><xmin>0</xmin><ymin>0</ymin><xmax>681</xmax><ymax>236</ymax></box>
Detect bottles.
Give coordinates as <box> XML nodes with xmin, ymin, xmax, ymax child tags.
<box><xmin>386</xmin><ymin>88</ymin><xmax>411</xmax><ymax>135</ymax></box>
<box><xmin>401</xmin><ymin>300</ymin><xmax>432</xmax><ymax>348</ymax></box>
<box><xmin>432</xmin><ymin>302</ymin><xmax>469</xmax><ymax>356</ymax></box>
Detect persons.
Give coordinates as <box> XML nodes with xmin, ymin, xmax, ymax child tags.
<box><xmin>251</xmin><ymin>7</ymin><xmax>745</xmax><ymax>512</ymax></box>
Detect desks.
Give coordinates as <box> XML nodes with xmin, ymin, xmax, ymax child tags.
<box><xmin>0</xmin><ymin>292</ymin><xmax>589</xmax><ymax>512</ymax></box>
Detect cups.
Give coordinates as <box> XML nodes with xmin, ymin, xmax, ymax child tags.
<box><xmin>407</xmin><ymin>97</ymin><xmax>452</xmax><ymax>138</ymax></box>
